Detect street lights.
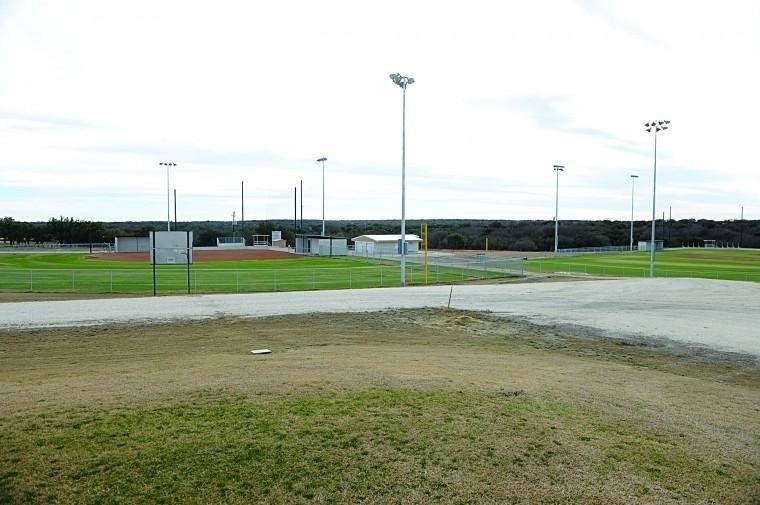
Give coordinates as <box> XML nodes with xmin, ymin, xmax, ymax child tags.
<box><xmin>552</xmin><ymin>164</ymin><xmax>565</xmax><ymax>254</ymax></box>
<box><xmin>644</xmin><ymin>119</ymin><xmax>672</xmax><ymax>277</ymax></box>
<box><xmin>159</xmin><ymin>159</ymin><xmax>177</xmax><ymax>233</ymax></box>
<box><xmin>629</xmin><ymin>174</ymin><xmax>640</xmax><ymax>250</ymax></box>
<box><xmin>388</xmin><ymin>73</ymin><xmax>416</xmax><ymax>288</ymax></box>
<box><xmin>317</xmin><ymin>157</ymin><xmax>328</xmax><ymax>235</ymax></box>
